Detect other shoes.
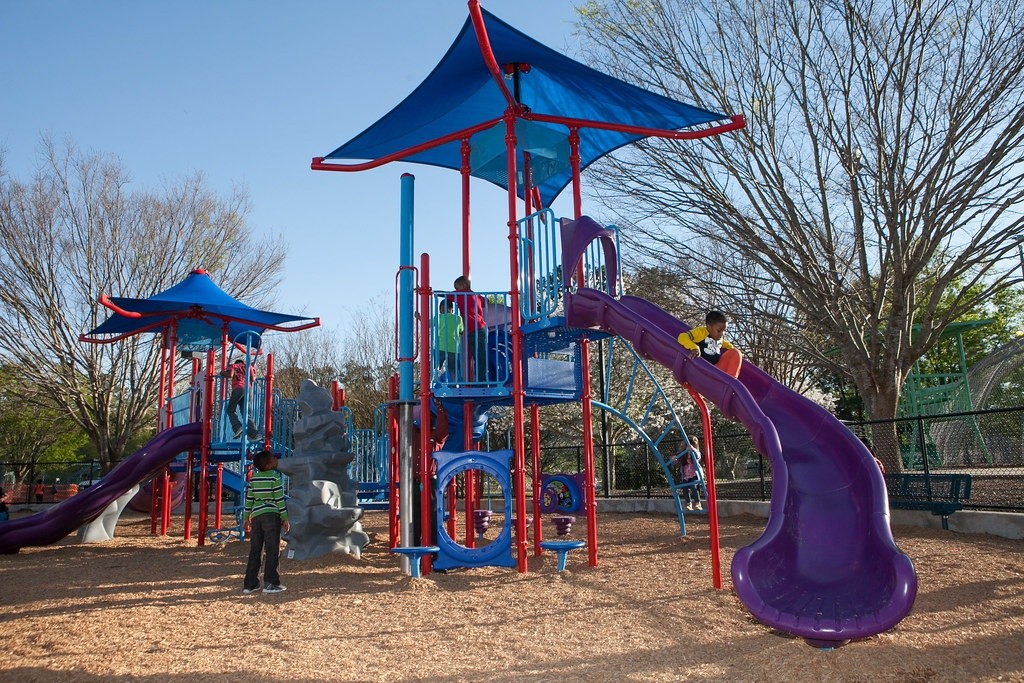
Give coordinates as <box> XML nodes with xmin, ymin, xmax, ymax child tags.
<box><xmin>233</xmin><ymin>427</ymin><xmax>243</xmax><ymax>439</ymax></box>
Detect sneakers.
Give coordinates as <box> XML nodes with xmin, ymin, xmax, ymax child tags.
<box><xmin>262</xmin><ymin>582</ymin><xmax>287</xmax><ymax>593</ymax></box>
<box><xmin>242</xmin><ymin>584</ymin><xmax>261</xmax><ymax>593</ymax></box>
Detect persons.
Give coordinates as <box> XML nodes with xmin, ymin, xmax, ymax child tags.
<box><xmin>861</xmin><ymin>436</ymin><xmax>885</xmax><ymax>474</ymax></box>
<box><xmin>677</xmin><ymin>309</ymin><xmax>742</xmax><ymax>380</ymax></box>
<box><xmin>51</xmin><ymin>481</ymin><xmax>58</xmax><ymax>501</ymax></box>
<box><xmin>415</xmin><ymin>299</ymin><xmax>464</xmax><ymax>388</ymax></box>
<box><xmin>216</xmin><ymin>358</ymin><xmax>263</xmax><ymax>442</ymax></box>
<box><xmin>34</xmin><ymin>479</ymin><xmax>47</xmax><ymax>503</ymax></box>
<box><xmin>668</xmin><ymin>436</ymin><xmax>704</xmax><ymax>511</ymax></box>
<box><xmin>241</xmin><ymin>450</ymin><xmax>288</xmax><ymax>593</ymax></box>
<box><xmin>433</xmin><ymin>275</ymin><xmax>490</xmax><ymax>388</ymax></box>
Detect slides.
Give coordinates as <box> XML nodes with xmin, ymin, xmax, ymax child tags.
<box><xmin>571</xmin><ymin>288</ymin><xmax>918</xmax><ymax>642</ymax></box>
<box><xmin>0</xmin><ymin>422</ymin><xmax>201</xmax><ymax>553</ymax></box>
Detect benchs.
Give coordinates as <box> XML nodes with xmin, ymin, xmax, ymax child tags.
<box><xmin>884</xmin><ymin>473</ymin><xmax>973</xmax><ymax>531</ymax></box>
